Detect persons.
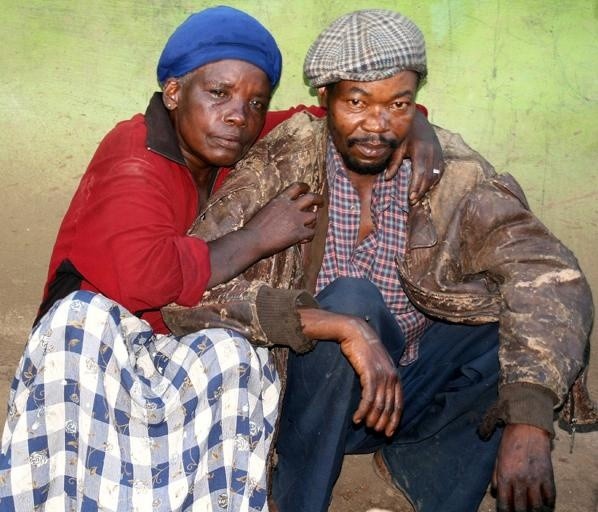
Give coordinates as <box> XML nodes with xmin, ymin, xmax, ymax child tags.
<box><xmin>161</xmin><ymin>9</ymin><xmax>595</xmax><ymax>511</ymax></box>
<box><xmin>1</xmin><ymin>6</ymin><xmax>446</xmax><ymax>510</ymax></box>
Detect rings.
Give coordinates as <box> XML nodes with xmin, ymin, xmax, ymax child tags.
<box><xmin>432</xmin><ymin>168</ymin><xmax>440</xmax><ymax>175</ymax></box>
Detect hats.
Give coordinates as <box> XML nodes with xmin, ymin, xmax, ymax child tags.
<box><xmin>156</xmin><ymin>5</ymin><xmax>283</xmax><ymax>92</ymax></box>
<box><xmin>302</xmin><ymin>7</ymin><xmax>428</xmax><ymax>89</ymax></box>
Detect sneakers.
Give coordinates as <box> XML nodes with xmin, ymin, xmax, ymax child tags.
<box><xmin>370</xmin><ymin>446</ymin><xmax>419</xmax><ymax>512</ymax></box>
